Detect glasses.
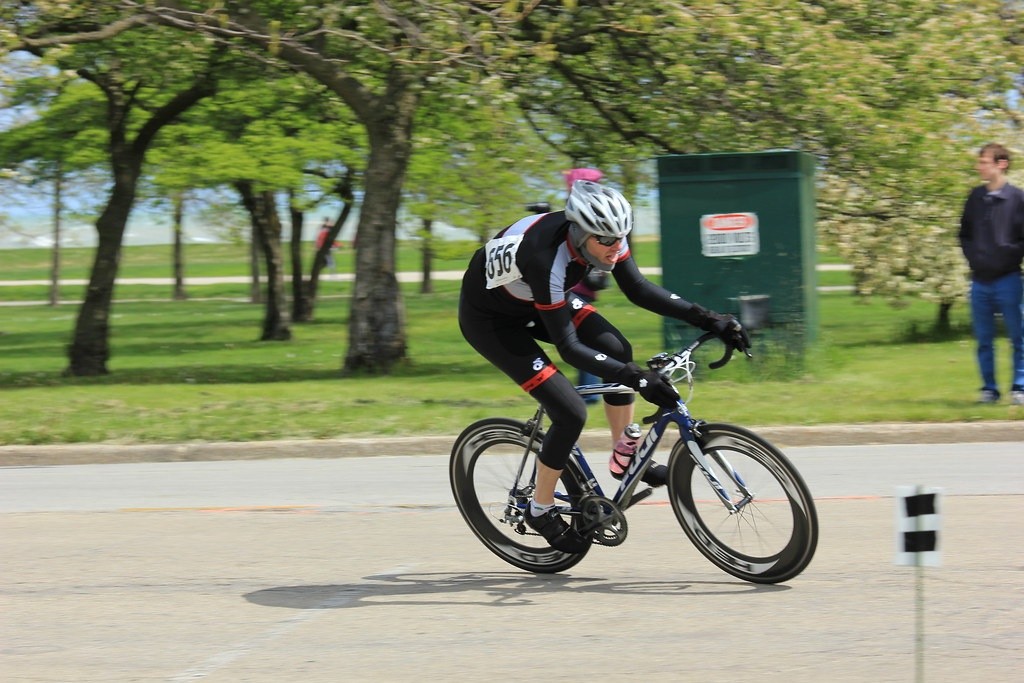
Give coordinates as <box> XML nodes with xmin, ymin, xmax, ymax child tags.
<box><xmin>592</xmin><ymin>234</ymin><xmax>623</xmax><ymax>246</ymax></box>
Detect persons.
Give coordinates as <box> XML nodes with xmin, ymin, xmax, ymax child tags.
<box><xmin>458</xmin><ymin>178</ymin><xmax>752</xmax><ymax>554</ymax></box>
<box><xmin>566</xmin><ymin>167</ymin><xmax>611</xmax><ymax>404</ymax></box>
<box><xmin>957</xmin><ymin>144</ymin><xmax>1024</xmax><ymax>405</ymax></box>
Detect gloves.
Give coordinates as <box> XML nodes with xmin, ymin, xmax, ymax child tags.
<box><xmin>690</xmin><ymin>304</ymin><xmax>752</xmax><ymax>352</ymax></box>
<box><xmin>619</xmin><ymin>361</ymin><xmax>682</xmax><ymax>410</ymax></box>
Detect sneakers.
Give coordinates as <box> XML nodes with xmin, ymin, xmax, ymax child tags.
<box><xmin>523</xmin><ymin>499</ymin><xmax>590</xmax><ymax>553</ymax></box>
<box><xmin>1010</xmin><ymin>385</ymin><xmax>1024</xmax><ymax>405</ymax></box>
<box><xmin>974</xmin><ymin>389</ymin><xmax>998</xmax><ymax>404</ymax></box>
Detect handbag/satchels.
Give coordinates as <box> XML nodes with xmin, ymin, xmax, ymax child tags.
<box><xmin>611</xmin><ymin>457</ymin><xmax>670</xmax><ymax>488</ymax></box>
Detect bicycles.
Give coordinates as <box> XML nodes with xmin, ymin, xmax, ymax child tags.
<box><xmin>449</xmin><ymin>334</ymin><xmax>820</xmax><ymax>584</ymax></box>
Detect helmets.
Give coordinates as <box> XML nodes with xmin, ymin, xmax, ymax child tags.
<box><xmin>564</xmin><ymin>180</ymin><xmax>634</xmax><ymax>239</ymax></box>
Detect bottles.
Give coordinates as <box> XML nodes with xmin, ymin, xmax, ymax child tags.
<box><xmin>609</xmin><ymin>422</ymin><xmax>642</xmax><ymax>474</ymax></box>
